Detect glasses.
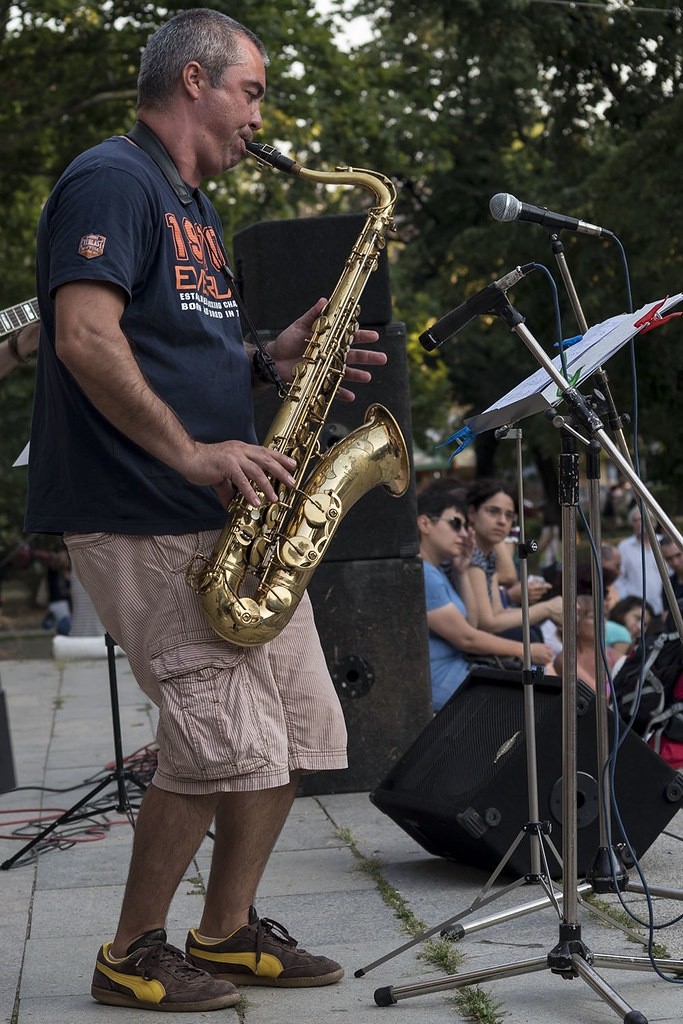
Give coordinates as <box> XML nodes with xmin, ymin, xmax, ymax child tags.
<box><xmin>428</xmin><ymin>514</ymin><xmax>467</xmax><ymax>531</ymax></box>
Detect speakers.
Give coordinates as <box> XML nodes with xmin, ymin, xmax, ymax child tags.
<box><xmin>371</xmin><ymin>668</ymin><xmax>683</xmax><ymax>879</ymax></box>
<box><xmin>233</xmin><ymin>217</ymin><xmax>436</xmax><ymax>796</ymax></box>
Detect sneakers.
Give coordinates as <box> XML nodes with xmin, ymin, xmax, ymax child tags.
<box><xmin>179</xmin><ymin>920</ymin><xmax>343</xmax><ymax>988</ymax></box>
<box><xmin>87</xmin><ymin>925</ymin><xmax>241</xmax><ymax>1011</ymax></box>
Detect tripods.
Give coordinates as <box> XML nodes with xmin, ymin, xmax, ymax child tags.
<box><xmin>1</xmin><ymin>630</ymin><xmax>215</xmax><ymax>872</ymax></box>
<box><xmin>355</xmin><ymin>235</ymin><xmax>682</xmax><ymax>1024</ymax></box>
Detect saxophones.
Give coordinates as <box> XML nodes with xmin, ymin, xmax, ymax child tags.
<box><xmin>196</xmin><ymin>141</ymin><xmax>410</xmax><ymax>648</ymax></box>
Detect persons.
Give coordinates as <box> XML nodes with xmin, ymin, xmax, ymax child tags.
<box><xmin>18</xmin><ymin>532</ymin><xmax>76</xmax><ymax>629</ymax></box>
<box><xmin>20</xmin><ymin>7</ymin><xmax>391</xmax><ymax>1012</ymax></box>
<box><xmin>416</xmin><ymin>475</ymin><xmax>683</xmax><ymax>777</ymax></box>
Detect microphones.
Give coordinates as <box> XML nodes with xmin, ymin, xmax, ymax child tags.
<box><xmin>490</xmin><ymin>192</ymin><xmax>613</xmax><ymax>238</ymax></box>
<box><xmin>419</xmin><ymin>261</ymin><xmax>536</xmax><ymax>352</ymax></box>
